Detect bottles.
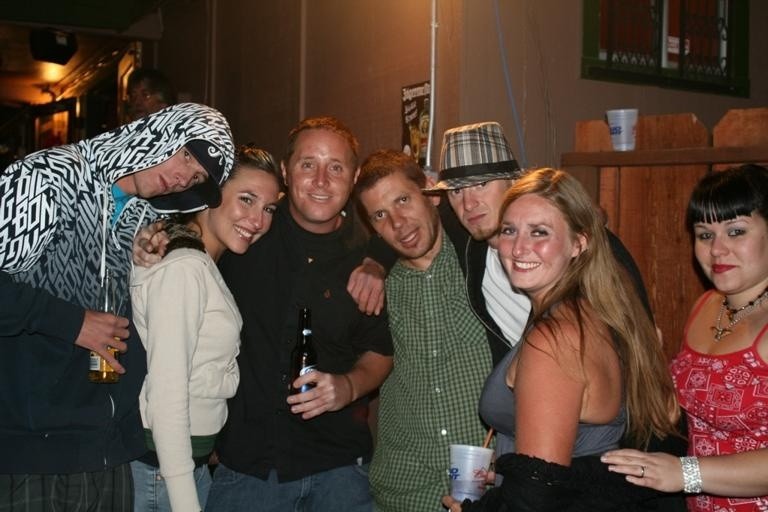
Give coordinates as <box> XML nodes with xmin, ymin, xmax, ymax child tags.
<box><xmin>289</xmin><ymin>303</ymin><xmax>317</xmax><ymax>415</ymax></box>
<box><xmin>418</xmin><ymin>99</ymin><xmax>430</xmax><ymax>166</ymax></box>
<box><xmin>408</xmin><ymin>124</ymin><xmax>419</xmax><ymax>163</ymax></box>
<box><xmin>88</xmin><ymin>269</ymin><xmax>119</xmax><ymax>383</ymax></box>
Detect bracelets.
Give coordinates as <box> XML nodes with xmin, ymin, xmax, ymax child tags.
<box><xmin>344</xmin><ymin>374</ymin><xmax>354</xmax><ymax>404</ymax></box>
<box><xmin>682</xmin><ymin>456</ymin><xmax>702</xmax><ymax>493</ymax></box>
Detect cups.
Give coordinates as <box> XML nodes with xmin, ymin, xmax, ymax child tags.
<box><xmin>605</xmin><ymin>108</ymin><xmax>639</xmax><ymax>151</ymax></box>
<box><xmin>449</xmin><ymin>444</ymin><xmax>495</xmax><ymax>502</ymax></box>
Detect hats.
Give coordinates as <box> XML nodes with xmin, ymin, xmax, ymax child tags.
<box><xmin>420</xmin><ymin>120</ymin><xmax>529</xmax><ymax>192</ymax></box>
<box><xmin>186</xmin><ymin>140</ymin><xmax>227</xmax><ymax>209</ymax></box>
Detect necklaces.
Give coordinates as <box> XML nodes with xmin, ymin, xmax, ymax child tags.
<box><xmin>711</xmin><ymin>294</ymin><xmax>764</xmax><ymax>341</ymax></box>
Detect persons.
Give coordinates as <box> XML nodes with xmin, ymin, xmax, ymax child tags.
<box><xmin>130</xmin><ymin>148</ymin><xmax>279</xmax><ymax>512</ymax></box>
<box><xmin>444</xmin><ymin>168</ymin><xmax>680</xmax><ymax>512</ymax></box>
<box><xmin>0</xmin><ymin>102</ymin><xmax>235</xmax><ymax>512</ymax></box>
<box><xmin>126</xmin><ymin>63</ymin><xmax>175</xmax><ymax>118</ymax></box>
<box><xmin>353</xmin><ymin>150</ymin><xmax>498</xmax><ymax>512</ymax></box>
<box><xmin>347</xmin><ymin>122</ymin><xmax>657</xmax><ymax>369</ymax></box>
<box><xmin>600</xmin><ymin>163</ymin><xmax>764</xmax><ymax>512</ymax></box>
<box><xmin>131</xmin><ymin>119</ymin><xmax>396</xmax><ymax>512</ymax></box>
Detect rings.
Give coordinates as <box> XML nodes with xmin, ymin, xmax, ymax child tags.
<box><xmin>639</xmin><ymin>466</ymin><xmax>645</xmax><ymax>475</ymax></box>
